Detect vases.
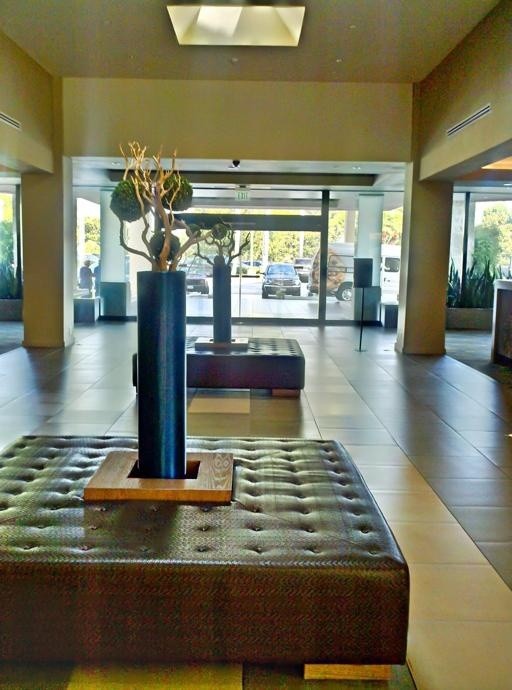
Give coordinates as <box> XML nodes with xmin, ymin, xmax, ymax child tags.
<box><xmin>136</xmin><ymin>271</ymin><xmax>188</xmax><ymax>479</ymax></box>
<box><xmin>213</xmin><ymin>262</ymin><xmax>232</xmax><ymax>344</ymax></box>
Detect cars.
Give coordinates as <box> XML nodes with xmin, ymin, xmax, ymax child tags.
<box><xmin>261</xmin><ymin>259</ymin><xmax>311</xmax><ymax>298</ymax></box>
<box><xmin>181</xmin><ymin>264</ymin><xmax>209</xmax><ymax>295</ymax></box>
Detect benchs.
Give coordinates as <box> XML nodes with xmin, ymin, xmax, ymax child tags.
<box><xmin>130</xmin><ymin>335</ymin><xmax>306</xmax><ymax>399</ymax></box>
<box><xmin>2</xmin><ymin>433</ymin><xmax>411</xmax><ymax>663</ymax></box>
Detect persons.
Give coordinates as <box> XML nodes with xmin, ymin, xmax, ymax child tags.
<box><xmin>79</xmin><ymin>260</ymin><xmax>95</xmax><ymax>298</ymax></box>
<box><xmin>94</xmin><ymin>260</ymin><xmax>100</xmax><ymax>296</ymax></box>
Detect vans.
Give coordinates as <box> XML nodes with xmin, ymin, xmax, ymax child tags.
<box><xmin>306</xmin><ymin>242</ymin><xmax>402</xmax><ymax>301</ymax></box>
<box><xmin>238</xmin><ymin>261</ymin><xmax>262</xmax><ymax>269</ymax></box>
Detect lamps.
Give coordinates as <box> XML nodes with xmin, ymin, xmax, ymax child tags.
<box><xmin>352</xmin><ymin>255</ymin><xmax>373</xmax><ymax>351</ymax></box>
